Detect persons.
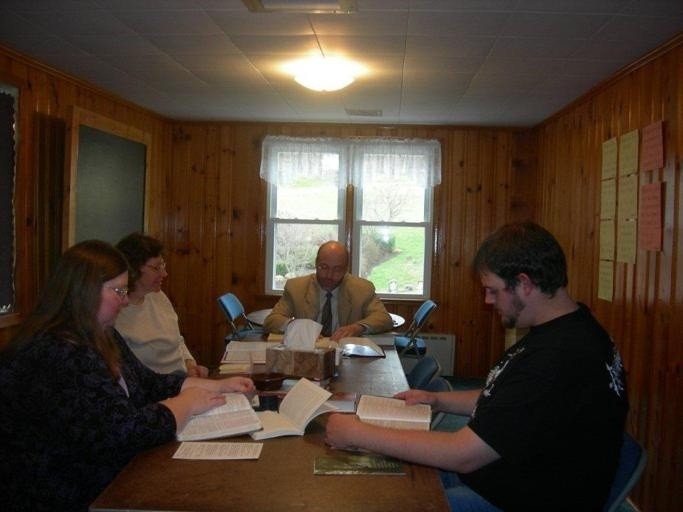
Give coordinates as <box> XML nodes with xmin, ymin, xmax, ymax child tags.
<box><xmin>110</xmin><ymin>233</ymin><xmax>210</xmax><ymax>386</ymax></box>
<box><xmin>323</xmin><ymin>220</ymin><xmax>628</xmax><ymax>512</ymax></box>
<box><xmin>261</xmin><ymin>240</ymin><xmax>394</xmax><ymax>344</ymax></box>
<box><xmin>2</xmin><ymin>238</ymin><xmax>257</xmax><ymax>509</ymax></box>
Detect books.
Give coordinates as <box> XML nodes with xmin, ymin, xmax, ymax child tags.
<box><xmin>313</xmin><ymin>336</ymin><xmax>385</xmax><ymax>358</ymax></box>
<box><xmin>356</xmin><ymin>393</ymin><xmax>432</xmax><ymax>435</ymax></box>
<box><xmin>247</xmin><ymin>377</ymin><xmax>338</xmax><ymax>442</ymax></box>
<box><xmin>312</xmin><ymin>450</ymin><xmax>407</xmax><ymax>477</ymax></box>
<box><xmin>172</xmin><ymin>391</ymin><xmax>264</xmax><ymax>442</ymax></box>
<box><xmin>218</xmin><ymin>340</ymin><xmax>282</xmax><ymax>375</ymax></box>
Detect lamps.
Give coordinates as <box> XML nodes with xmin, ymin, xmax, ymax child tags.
<box><xmin>286</xmin><ymin>51</ymin><xmax>362</xmax><ymax>93</ymax></box>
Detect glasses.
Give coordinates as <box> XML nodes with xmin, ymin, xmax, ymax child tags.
<box><xmin>143</xmin><ymin>262</ymin><xmax>166</xmax><ymax>273</ymax></box>
<box><xmin>102</xmin><ymin>286</ymin><xmax>129</xmax><ymax>297</ymax></box>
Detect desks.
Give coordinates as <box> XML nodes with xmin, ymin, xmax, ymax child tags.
<box><xmin>246</xmin><ymin>308</ymin><xmax>407</xmax><ymax>329</ymax></box>
<box><xmin>85</xmin><ymin>330</ymin><xmax>454</xmax><ymax>511</ymax></box>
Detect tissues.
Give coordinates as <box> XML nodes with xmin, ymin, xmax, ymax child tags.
<box><xmin>265</xmin><ymin>319</ymin><xmax>336</xmax><ymax>381</ymax></box>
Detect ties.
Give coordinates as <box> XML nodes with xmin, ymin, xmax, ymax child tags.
<box><xmin>320</xmin><ymin>292</ymin><xmax>332</xmax><ymax>338</ymax></box>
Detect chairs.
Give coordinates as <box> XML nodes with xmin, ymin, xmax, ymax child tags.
<box><xmin>216</xmin><ymin>292</ymin><xmax>265</xmax><ymax>344</ymax></box>
